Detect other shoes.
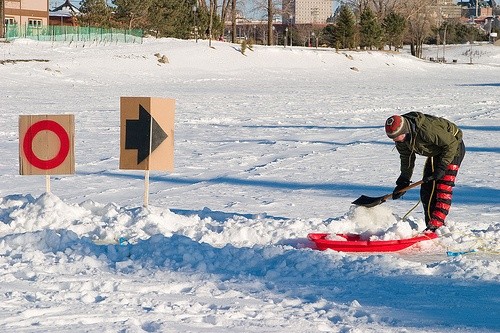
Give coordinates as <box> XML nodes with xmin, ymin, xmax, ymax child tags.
<box><xmin>424</xmin><ymin>226</ymin><xmax>444</xmax><ymax>232</ymax></box>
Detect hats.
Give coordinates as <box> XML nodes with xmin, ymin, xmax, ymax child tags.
<box><xmin>385</xmin><ymin>114</ymin><xmax>406</xmax><ymax>139</ymax></box>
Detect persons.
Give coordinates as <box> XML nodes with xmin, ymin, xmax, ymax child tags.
<box><xmin>385</xmin><ymin>111</ymin><xmax>467</xmax><ymax>235</ymax></box>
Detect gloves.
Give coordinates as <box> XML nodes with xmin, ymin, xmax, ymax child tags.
<box><xmin>432</xmin><ymin>163</ymin><xmax>446</xmax><ymax>179</ymax></box>
<box><xmin>392</xmin><ymin>177</ymin><xmax>410</xmax><ymax>199</ymax></box>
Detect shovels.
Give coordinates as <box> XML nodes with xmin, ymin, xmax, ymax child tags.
<box><xmin>351</xmin><ymin>176</ymin><xmax>435</xmax><ymax>208</ymax></box>
